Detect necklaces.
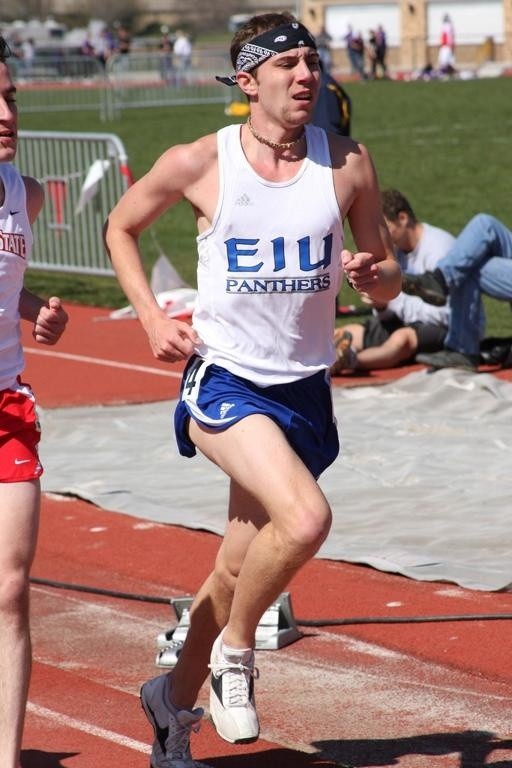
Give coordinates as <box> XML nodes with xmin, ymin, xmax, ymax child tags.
<box><xmin>246</xmin><ymin>115</ymin><xmax>307</xmax><ymax>151</ymax></box>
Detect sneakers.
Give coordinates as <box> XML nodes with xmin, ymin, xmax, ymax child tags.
<box><xmin>208</xmin><ymin>623</ymin><xmax>262</xmax><ymax>746</ymax></box>
<box><xmin>397</xmin><ymin>268</ymin><xmax>448</xmax><ymax>308</ymax></box>
<box><xmin>138</xmin><ymin>668</ymin><xmax>206</xmax><ymax>768</ymax></box>
<box><xmin>412</xmin><ymin>350</ymin><xmax>480</xmax><ymax>374</ymax></box>
<box><xmin>328</xmin><ymin>327</ymin><xmax>360</xmax><ymax>376</ymax></box>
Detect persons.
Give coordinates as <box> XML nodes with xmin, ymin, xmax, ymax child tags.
<box><xmin>329</xmin><ymin>188</ymin><xmax>455</xmax><ymax>378</ymax></box>
<box><xmin>403</xmin><ymin>210</ymin><xmax>511</xmax><ymax>372</ymax></box>
<box><xmin>100</xmin><ymin>9</ymin><xmax>404</xmax><ymax>768</ymax></box>
<box><xmin>1</xmin><ymin>38</ymin><xmax>71</xmax><ymax>768</ymax></box>
